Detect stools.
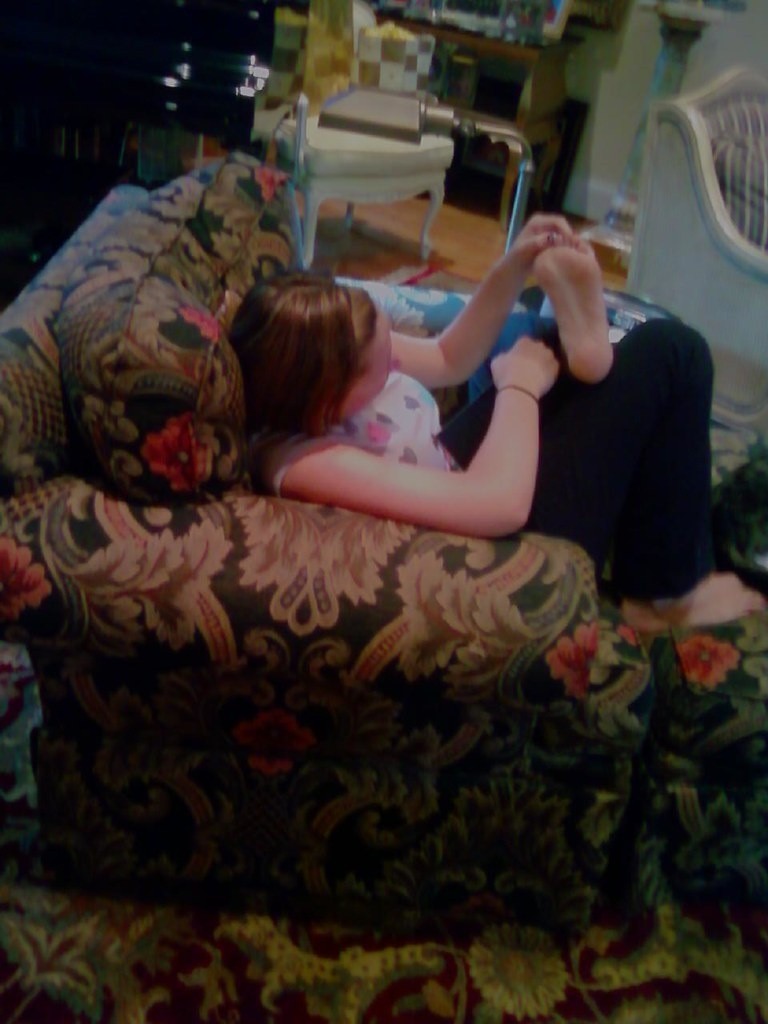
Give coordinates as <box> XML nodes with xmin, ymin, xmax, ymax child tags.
<box><xmin>271</xmin><ymin>116</ymin><xmax>458</xmax><ymax>268</ymax></box>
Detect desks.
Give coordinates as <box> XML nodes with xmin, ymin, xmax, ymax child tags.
<box><xmin>361</xmin><ymin>21</ymin><xmax>589</xmax><ymax>232</ymax></box>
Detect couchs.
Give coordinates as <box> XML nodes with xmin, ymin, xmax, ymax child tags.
<box><xmin>624</xmin><ymin>64</ymin><xmax>768</xmax><ymax>426</ymax></box>
<box><xmin>0</xmin><ymin>165</ymin><xmax>768</xmax><ymax>1024</ymax></box>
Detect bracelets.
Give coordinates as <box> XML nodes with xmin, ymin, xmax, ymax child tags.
<box><xmin>497</xmin><ymin>385</ymin><xmax>539</xmax><ymax>403</ymax></box>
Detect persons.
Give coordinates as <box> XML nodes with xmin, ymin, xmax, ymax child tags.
<box><xmin>228</xmin><ymin>213</ymin><xmax>765</xmax><ymax>637</ymax></box>
